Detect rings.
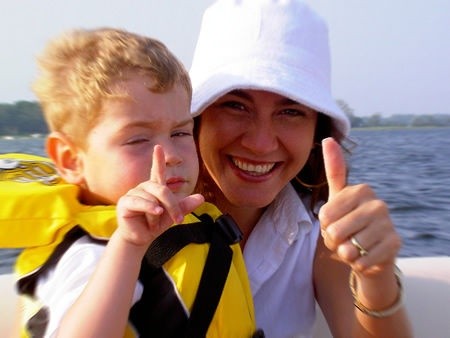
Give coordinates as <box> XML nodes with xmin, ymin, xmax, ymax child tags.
<box><xmin>351</xmin><ymin>236</ymin><xmax>369</xmax><ymax>256</ymax></box>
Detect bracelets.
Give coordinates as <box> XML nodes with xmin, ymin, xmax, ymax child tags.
<box><xmin>350</xmin><ymin>266</ymin><xmax>407</xmax><ymax>318</ymax></box>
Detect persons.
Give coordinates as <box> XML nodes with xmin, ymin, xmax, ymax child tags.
<box><xmin>45</xmin><ymin>0</ymin><xmax>415</xmax><ymax>338</ymax></box>
<box><xmin>13</xmin><ymin>29</ymin><xmax>264</xmax><ymax>338</ymax></box>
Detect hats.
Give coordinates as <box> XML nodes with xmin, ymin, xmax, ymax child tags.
<box><xmin>188</xmin><ymin>0</ymin><xmax>350</xmax><ymax>143</ymax></box>
